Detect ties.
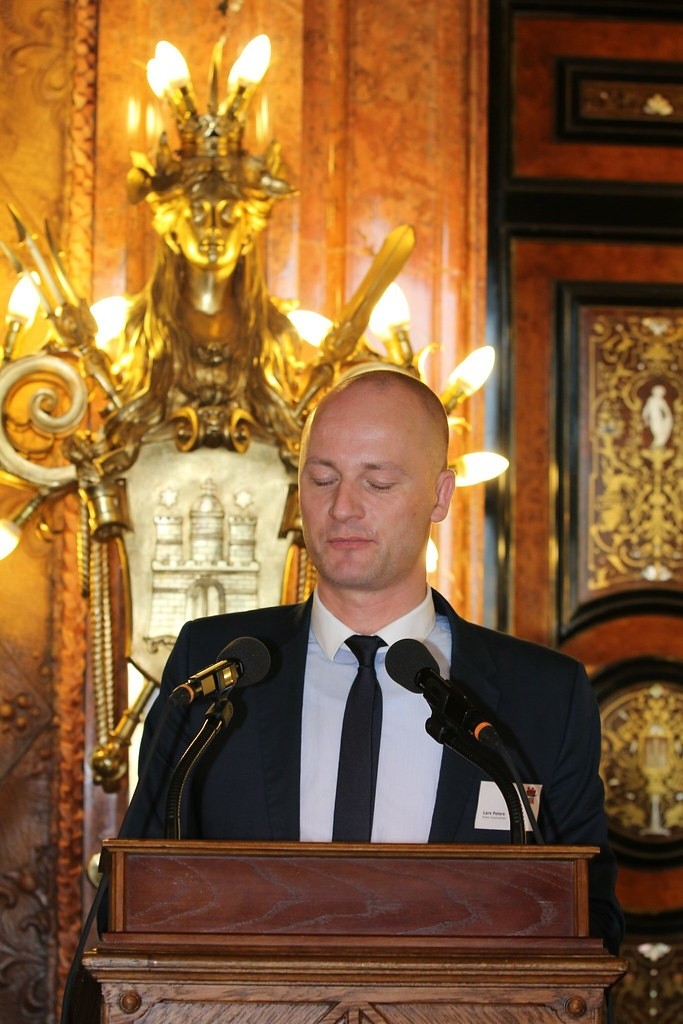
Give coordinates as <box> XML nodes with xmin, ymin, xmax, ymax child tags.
<box><xmin>331</xmin><ymin>634</ymin><xmax>389</xmax><ymax>843</ymax></box>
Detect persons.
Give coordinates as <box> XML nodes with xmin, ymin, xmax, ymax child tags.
<box><xmin>96</xmin><ymin>366</ymin><xmax>627</xmax><ymax>944</ymax></box>
<box><xmin>109</xmin><ymin>129</ymin><xmax>317</xmax><ymax>454</ymax></box>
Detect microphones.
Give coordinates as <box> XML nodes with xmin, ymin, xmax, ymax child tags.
<box><xmin>167</xmin><ymin>636</ymin><xmax>270</xmax><ymax>708</ymax></box>
<box><xmin>385</xmin><ymin>638</ymin><xmax>503</xmax><ymax>751</ymax></box>
